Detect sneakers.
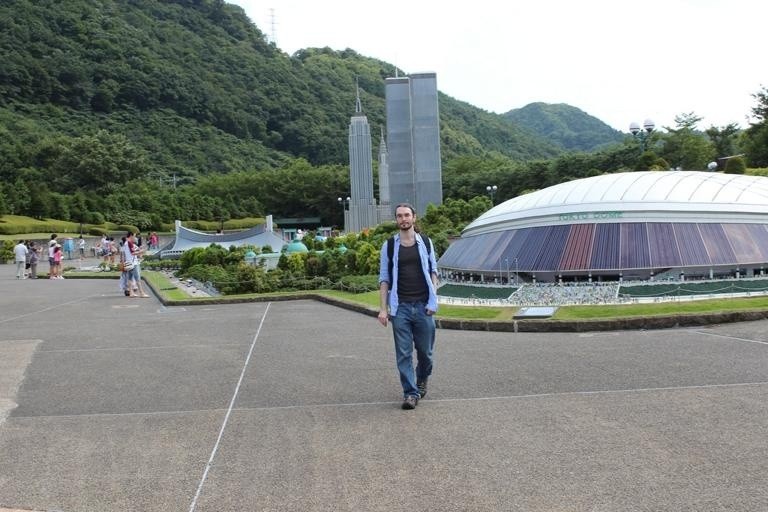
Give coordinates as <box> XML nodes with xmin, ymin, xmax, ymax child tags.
<box><xmin>50</xmin><ymin>275</ymin><xmax>64</xmax><ymax>280</ymax></box>
<box><xmin>402</xmin><ymin>377</ymin><xmax>427</xmax><ymax>409</ymax></box>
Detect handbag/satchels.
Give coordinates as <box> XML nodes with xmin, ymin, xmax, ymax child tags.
<box><xmin>118</xmin><ymin>261</ymin><xmax>135</xmax><ymax>271</ymax></box>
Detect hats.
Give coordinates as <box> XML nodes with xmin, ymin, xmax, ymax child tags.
<box><xmin>50</xmin><ymin>240</ymin><xmax>61</xmax><ymax>247</ymax></box>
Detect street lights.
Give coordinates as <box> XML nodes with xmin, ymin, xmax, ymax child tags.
<box><xmin>628</xmin><ymin>119</ymin><xmax>654</xmax><ymax>155</ymax></box>
<box><xmin>337</xmin><ymin>197</ymin><xmax>349</xmax><ymax>215</ymax></box>
<box><xmin>486</xmin><ymin>186</ymin><xmax>498</xmax><ymax>205</ymax></box>
<box><xmin>706</xmin><ymin>161</ymin><xmax>716</xmax><ymax>172</ymax></box>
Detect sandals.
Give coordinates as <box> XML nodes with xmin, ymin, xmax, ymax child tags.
<box><xmin>124</xmin><ymin>287</ymin><xmax>149</xmax><ymax>297</ymax></box>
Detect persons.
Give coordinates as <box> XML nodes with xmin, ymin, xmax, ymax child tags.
<box><xmin>378</xmin><ymin>203</ymin><xmax>439</xmax><ymax>410</ymax></box>
<box><xmin>13</xmin><ymin>234</ymin><xmax>85</xmax><ymax>281</ymax></box>
<box><xmin>102</xmin><ymin>232</ymin><xmax>158</xmax><ymax>298</ymax></box>
